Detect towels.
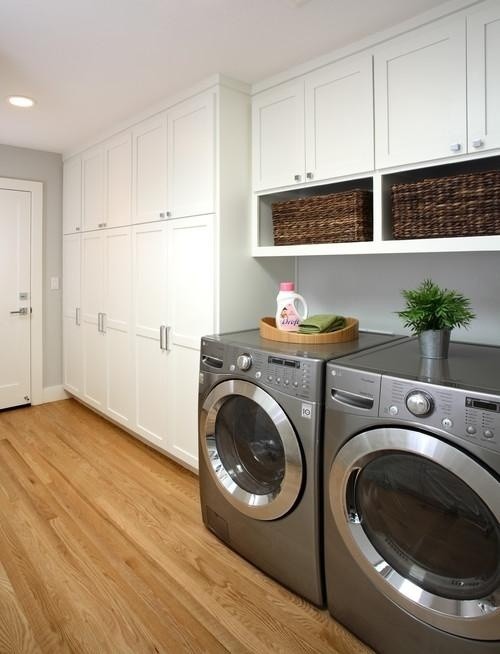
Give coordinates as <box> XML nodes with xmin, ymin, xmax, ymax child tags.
<box><xmin>299</xmin><ymin>312</ymin><xmax>346</xmax><ymax>334</ymax></box>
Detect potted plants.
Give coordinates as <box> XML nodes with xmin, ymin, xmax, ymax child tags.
<box><xmin>393</xmin><ymin>276</ymin><xmax>477</xmax><ymax>359</ymax></box>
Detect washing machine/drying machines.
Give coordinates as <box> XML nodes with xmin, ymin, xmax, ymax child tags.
<box><xmin>198</xmin><ymin>328</ymin><xmax>409</xmax><ymax>608</ymax></box>
<box><xmin>323</xmin><ymin>338</ymin><xmax>500</xmax><ymax>654</ymax></box>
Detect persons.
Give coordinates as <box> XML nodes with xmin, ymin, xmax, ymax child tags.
<box><xmin>280</xmin><ymin>307</ymin><xmax>288</xmax><ymax>324</ymax></box>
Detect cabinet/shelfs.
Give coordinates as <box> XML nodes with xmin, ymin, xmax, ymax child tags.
<box><xmin>63</xmin><ymin>146</ymin><xmax>83</xmax><ymax>403</ymax></box>
<box><xmin>84</xmin><ymin>132</ymin><xmax>133</xmax><ymax>437</ymax></box>
<box><xmin>249</xmin><ymin>35</ymin><xmax>375</xmax><ymax>256</ymax></box>
<box><xmin>375</xmin><ymin>0</ymin><xmax>500</xmax><ymax>256</ymax></box>
<box><xmin>133</xmin><ymin>85</ymin><xmax>296</xmax><ymax>476</ymax></box>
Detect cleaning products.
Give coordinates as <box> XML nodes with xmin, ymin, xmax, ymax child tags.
<box><xmin>276</xmin><ymin>282</ymin><xmax>308</xmax><ymax>331</ymax></box>
